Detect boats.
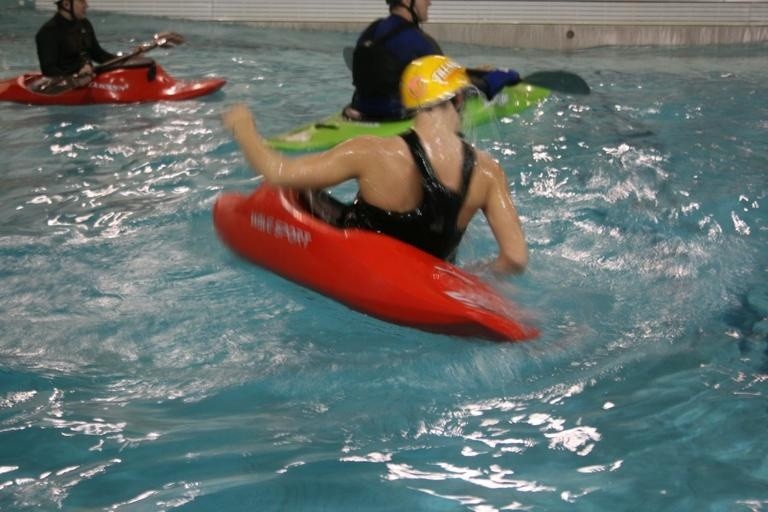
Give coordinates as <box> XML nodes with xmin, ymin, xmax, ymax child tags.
<box><xmin>206</xmin><ymin>175</ymin><xmax>545</xmax><ymax>352</ymax></box>
<box><xmin>0</xmin><ymin>56</ymin><xmax>230</xmax><ymax>109</ymax></box>
<box><xmin>257</xmin><ymin>77</ymin><xmax>554</xmax><ymax>153</ymax></box>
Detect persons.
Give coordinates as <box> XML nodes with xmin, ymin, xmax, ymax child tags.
<box><xmin>350</xmin><ymin>0</ymin><xmax>445</xmax><ymax>121</ymax></box>
<box><xmin>34</xmin><ymin>0</ymin><xmax>145</xmax><ymax>94</ymax></box>
<box><xmin>221</xmin><ymin>52</ymin><xmax>529</xmax><ymax>282</ymax></box>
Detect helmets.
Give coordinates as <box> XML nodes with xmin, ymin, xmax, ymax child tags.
<box><xmin>399</xmin><ymin>54</ymin><xmax>472</xmax><ymax>112</ymax></box>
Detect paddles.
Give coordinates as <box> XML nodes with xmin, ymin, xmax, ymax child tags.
<box><xmin>343</xmin><ymin>45</ymin><xmax>591</xmax><ymax>95</ymax></box>
<box><xmin>27</xmin><ymin>32</ymin><xmax>184</xmax><ymax>97</ymax></box>
